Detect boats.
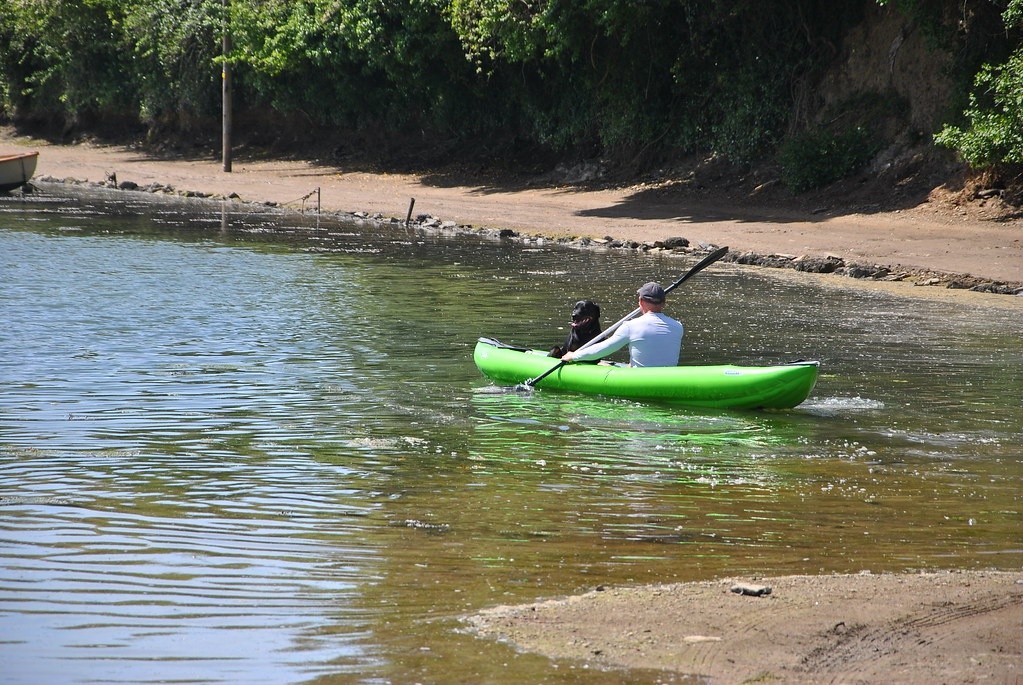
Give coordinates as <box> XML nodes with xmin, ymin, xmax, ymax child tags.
<box><xmin>0</xmin><ymin>151</ymin><xmax>40</xmax><ymax>194</ymax></box>
<box><xmin>474</xmin><ymin>336</ymin><xmax>821</xmax><ymax>411</ymax></box>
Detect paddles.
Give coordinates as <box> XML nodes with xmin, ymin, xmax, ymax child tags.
<box><xmin>528</xmin><ymin>244</ymin><xmax>731</xmax><ymax>387</ymax></box>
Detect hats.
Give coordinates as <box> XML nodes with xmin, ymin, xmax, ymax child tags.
<box><xmin>637</xmin><ymin>282</ymin><xmax>665</xmax><ymax>305</ymax></box>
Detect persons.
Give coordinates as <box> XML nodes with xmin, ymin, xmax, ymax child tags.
<box><xmin>561</xmin><ymin>282</ymin><xmax>684</xmax><ymax>368</ymax></box>
